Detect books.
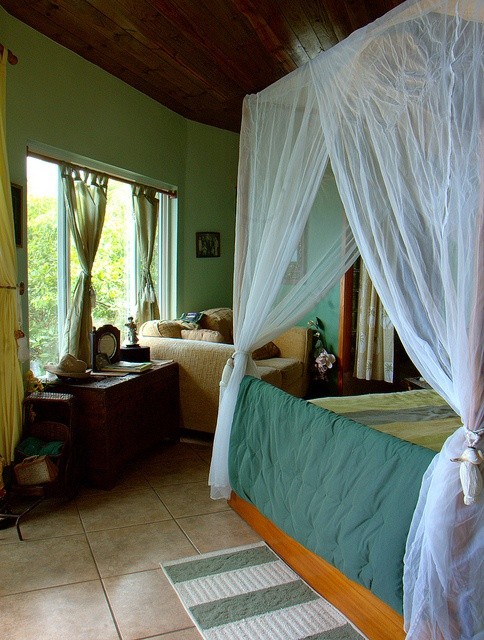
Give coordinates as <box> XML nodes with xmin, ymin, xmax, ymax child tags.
<box><xmin>91</xmin><ymin>361</ymin><xmax>153</xmax><ymax>377</ymax></box>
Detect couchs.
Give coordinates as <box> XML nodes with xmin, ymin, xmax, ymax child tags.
<box><xmin>137</xmin><ymin>307</ymin><xmax>313</xmax><ymax>433</ymax></box>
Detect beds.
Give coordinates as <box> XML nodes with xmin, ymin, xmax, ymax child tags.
<box><xmin>224</xmin><ymin>376</ymin><xmax>484</xmax><ymax>639</ymax></box>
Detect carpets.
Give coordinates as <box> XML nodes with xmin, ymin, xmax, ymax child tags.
<box><xmin>158</xmin><ymin>539</ymin><xmax>370</xmax><ymax>638</ymax></box>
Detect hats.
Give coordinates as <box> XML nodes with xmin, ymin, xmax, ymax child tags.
<box><xmin>48</xmin><ymin>354</ymin><xmax>91</xmax><ymax>378</ymax></box>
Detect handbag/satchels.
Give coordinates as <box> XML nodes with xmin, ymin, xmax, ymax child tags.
<box><xmin>14</xmin><ymin>454</ymin><xmax>59</xmax><ymax>486</ymax></box>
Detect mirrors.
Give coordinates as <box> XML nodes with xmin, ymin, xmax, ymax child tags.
<box><xmin>98</xmin><ymin>333</ymin><xmax>118</xmax><ymax>359</ymax></box>
<box><xmin>91</xmin><ymin>326</ymin><xmax>120</xmax><ymax>373</ymax></box>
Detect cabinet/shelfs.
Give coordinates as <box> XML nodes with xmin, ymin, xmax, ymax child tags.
<box><xmin>38</xmin><ymin>360</ymin><xmax>180</xmax><ymax>490</ymax></box>
<box><xmin>404</xmin><ymin>376</ymin><xmax>431</xmax><ymax>390</ymax></box>
<box><xmin>337</xmin><ymin>215</ymin><xmax>407</xmax><ymax>394</ymax></box>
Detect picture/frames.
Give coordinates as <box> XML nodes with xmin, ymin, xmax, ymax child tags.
<box><xmin>10</xmin><ymin>181</ymin><xmax>23</xmax><ymax>247</ymax></box>
<box><xmin>195</xmin><ymin>231</ymin><xmax>220</xmax><ymax>258</ymax></box>
<box><xmin>278</xmin><ymin>220</ymin><xmax>303</xmax><ymax>285</ymax></box>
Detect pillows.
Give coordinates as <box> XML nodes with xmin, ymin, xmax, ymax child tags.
<box><xmin>181</xmin><ymin>329</ymin><xmax>223</xmax><ymax>343</ymax></box>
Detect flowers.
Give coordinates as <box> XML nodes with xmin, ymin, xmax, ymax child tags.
<box><xmin>308</xmin><ymin>319</ymin><xmax>338</xmax><ymax>381</ymax></box>
